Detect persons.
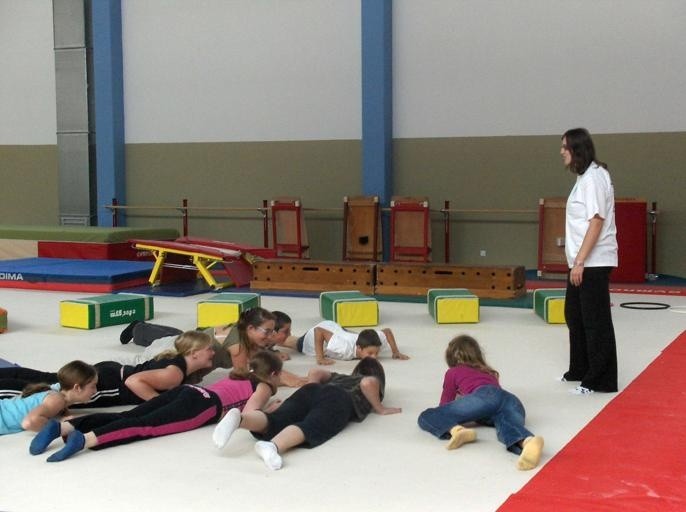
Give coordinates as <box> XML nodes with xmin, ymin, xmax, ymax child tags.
<box><xmin>119</xmin><ymin>306</ymin><xmax>309</xmax><ymax>388</ymax></box>
<box><xmin>28</xmin><ymin>352</ymin><xmax>283</xmax><ymax>463</ymax></box>
<box><xmin>560</xmin><ymin>128</ymin><xmax>620</xmax><ymax>394</ymax></box>
<box><xmin>0</xmin><ymin>330</ymin><xmax>215</xmax><ymax>411</ymax></box>
<box><xmin>0</xmin><ymin>360</ymin><xmax>99</xmax><ymax>434</ymax></box>
<box><xmin>120</xmin><ymin>311</ymin><xmax>292</xmax><ymax>362</ymax></box>
<box><xmin>211</xmin><ymin>355</ymin><xmax>402</xmax><ymax>473</ymax></box>
<box><xmin>280</xmin><ymin>319</ymin><xmax>411</xmax><ymax>365</ymax></box>
<box><xmin>417</xmin><ymin>334</ymin><xmax>544</xmax><ymax>471</ymax></box>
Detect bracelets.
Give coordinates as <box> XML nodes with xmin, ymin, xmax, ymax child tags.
<box><xmin>574</xmin><ymin>261</ymin><xmax>584</xmax><ymax>267</ymax></box>
